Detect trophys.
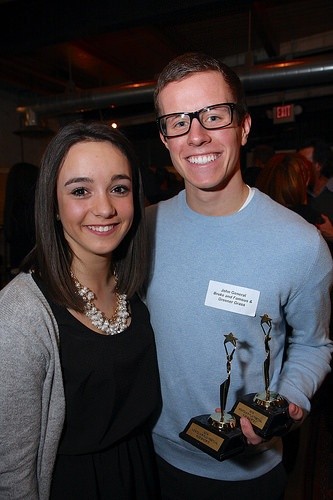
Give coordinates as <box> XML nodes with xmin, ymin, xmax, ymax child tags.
<box><xmin>227</xmin><ymin>314</ymin><xmax>294</xmax><ymax>441</ymax></box>
<box><xmin>179</xmin><ymin>333</ymin><xmax>250</xmax><ymax>463</ymax></box>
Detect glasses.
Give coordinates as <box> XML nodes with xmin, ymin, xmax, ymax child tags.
<box><xmin>154</xmin><ymin>103</ymin><xmax>240</xmax><ymax>139</ymax></box>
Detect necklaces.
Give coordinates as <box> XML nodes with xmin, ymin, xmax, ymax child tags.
<box><xmin>69</xmin><ymin>264</ymin><xmax>130</xmax><ymax>336</ymax></box>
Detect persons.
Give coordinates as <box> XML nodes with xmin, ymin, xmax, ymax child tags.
<box><xmin>2</xmin><ymin>126</ymin><xmax>333</xmax><ymax>284</ymax></box>
<box><xmin>0</xmin><ymin>121</ymin><xmax>163</xmax><ymax>500</ymax></box>
<box><xmin>145</xmin><ymin>51</ymin><xmax>333</xmax><ymax>500</ymax></box>
